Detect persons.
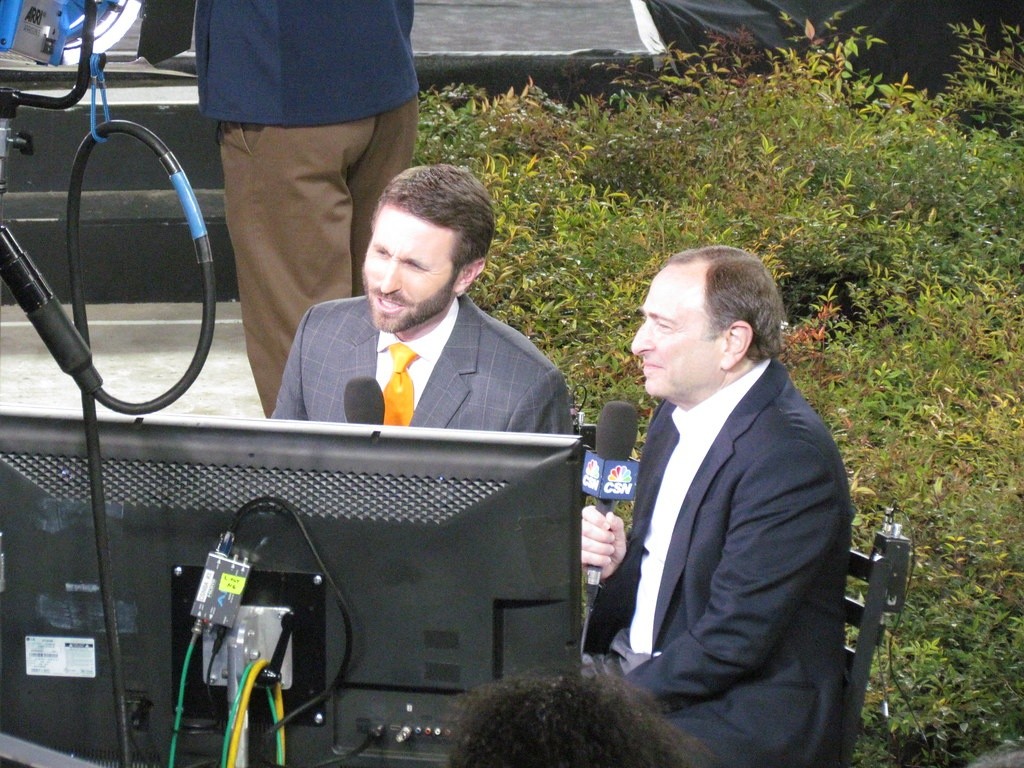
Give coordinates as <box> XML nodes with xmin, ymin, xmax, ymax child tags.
<box><xmin>582</xmin><ymin>247</ymin><xmax>853</xmax><ymax>767</ymax></box>
<box><xmin>451</xmin><ymin>672</ymin><xmax>711</xmax><ymax>768</ymax></box>
<box><xmin>194</xmin><ymin>0</ymin><xmax>418</xmax><ymax>418</ymax></box>
<box><xmin>269</xmin><ymin>166</ymin><xmax>573</xmax><ymax>433</ymax></box>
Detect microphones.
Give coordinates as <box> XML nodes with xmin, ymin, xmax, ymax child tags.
<box><xmin>344</xmin><ymin>375</ymin><xmax>385</xmax><ymax>424</ymax></box>
<box><xmin>581</xmin><ymin>401</ymin><xmax>639</xmax><ymax>607</ymax></box>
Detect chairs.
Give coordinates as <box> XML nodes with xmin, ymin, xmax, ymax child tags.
<box><xmin>817</xmin><ymin>547</ymin><xmax>896</xmax><ymax>768</ymax></box>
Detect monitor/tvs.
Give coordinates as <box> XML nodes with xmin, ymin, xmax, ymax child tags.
<box><xmin>0</xmin><ymin>410</ymin><xmax>586</xmax><ymax>768</ymax></box>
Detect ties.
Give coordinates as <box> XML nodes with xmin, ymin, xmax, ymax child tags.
<box><xmin>379</xmin><ymin>342</ymin><xmax>420</xmax><ymax>426</ymax></box>
